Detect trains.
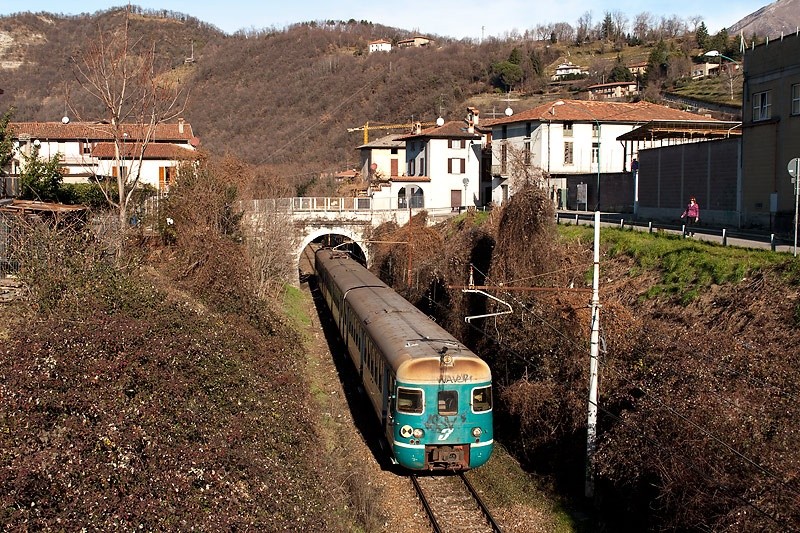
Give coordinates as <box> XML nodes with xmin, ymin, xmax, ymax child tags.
<box><xmin>314</xmin><ymin>246</ymin><xmax>495</xmax><ymax>474</ymax></box>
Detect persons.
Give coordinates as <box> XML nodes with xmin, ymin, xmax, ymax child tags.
<box><xmin>681</xmin><ymin>196</ymin><xmax>699</xmax><ymax>238</ymax></box>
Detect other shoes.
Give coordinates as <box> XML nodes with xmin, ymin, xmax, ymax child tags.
<box><xmin>685</xmin><ymin>235</ymin><xmax>689</xmax><ymax>238</ymax></box>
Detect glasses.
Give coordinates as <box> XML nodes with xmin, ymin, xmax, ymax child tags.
<box><xmin>691</xmin><ymin>200</ymin><xmax>694</xmax><ymax>201</ymax></box>
